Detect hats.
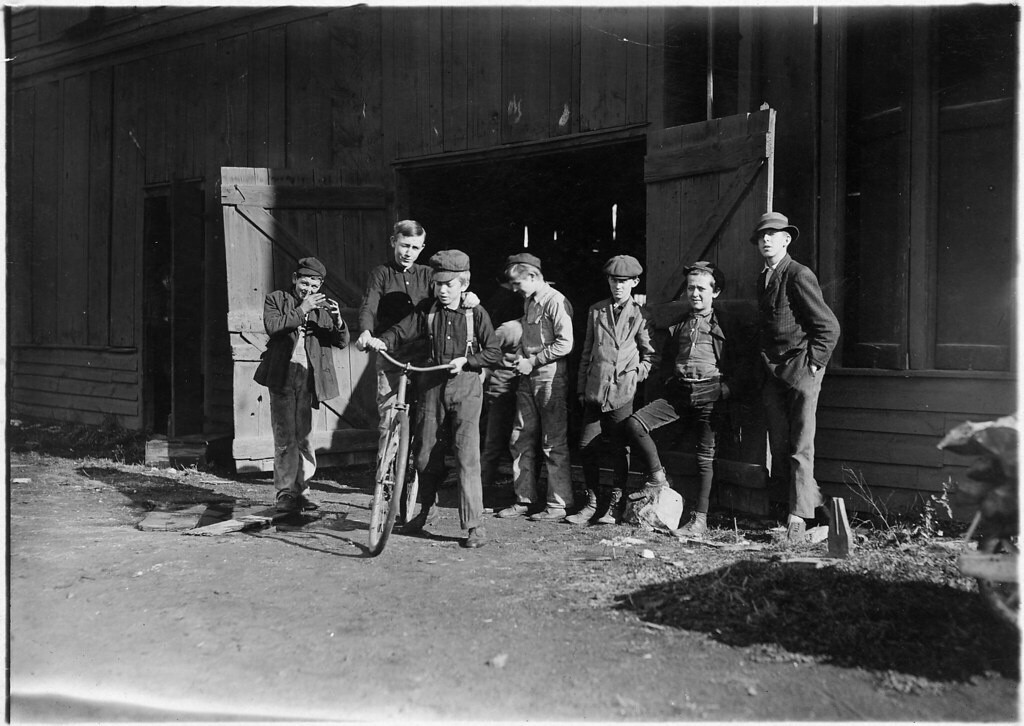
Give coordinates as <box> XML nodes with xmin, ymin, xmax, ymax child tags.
<box><xmin>749</xmin><ymin>212</ymin><xmax>799</xmax><ymax>246</ymax></box>
<box><xmin>505</xmin><ymin>253</ymin><xmax>542</xmax><ymax>270</ymax></box>
<box><xmin>682</xmin><ymin>261</ymin><xmax>726</xmax><ymax>294</ymax></box>
<box><xmin>296</xmin><ymin>257</ymin><xmax>327</xmax><ymax>280</ymax></box>
<box><xmin>602</xmin><ymin>255</ymin><xmax>644</xmax><ymax>280</ymax></box>
<box><xmin>430</xmin><ymin>249</ymin><xmax>470</xmax><ymax>282</ymax></box>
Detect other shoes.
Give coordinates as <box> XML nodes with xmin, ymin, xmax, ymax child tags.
<box><xmin>465</xmin><ymin>524</ymin><xmax>488</xmax><ymax>548</ymax></box>
<box><xmin>787</xmin><ymin>519</ymin><xmax>806</xmax><ymax>541</ymax></box>
<box><xmin>398</xmin><ymin>506</ymin><xmax>443</xmax><ymax>535</ymax></box>
<box><xmin>275</xmin><ymin>494</ymin><xmax>318</xmax><ymax>511</ymax></box>
<box><xmin>816</xmin><ymin>491</ymin><xmax>824</xmax><ymax>509</ymax></box>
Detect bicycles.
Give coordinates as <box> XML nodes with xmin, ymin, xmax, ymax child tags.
<box><xmin>356</xmin><ymin>343</ymin><xmax>455</xmax><ymax>557</ymax></box>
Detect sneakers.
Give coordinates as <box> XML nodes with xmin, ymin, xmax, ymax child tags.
<box><xmin>497</xmin><ymin>503</ymin><xmax>538</xmax><ymax>517</ymax></box>
<box><xmin>530</xmin><ymin>507</ymin><xmax>567</xmax><ymax>521</ymax></box>
<box><xmin>676</xmin><ymin>511</ymin><xmax>708</xmax><ymax>536</ymax></box>
<box><xmin>629</xmin><ymin>480</ymin><xmax>670</xmax><ymax>501</ymax></box>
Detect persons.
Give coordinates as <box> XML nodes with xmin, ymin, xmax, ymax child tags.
<box><xmin>359</xmin><ymin>219</ymin><xmax>481</xmax><ymax>482</ymax></box>
<box><xmin>626</xmin><ymin>260</ymin><xmax>735</xmax><ymax>536</ymax></box>
<box><xmin>729</xmin><ymin>212</ymin><xmax>841</xmax><ymax>541</ymax></box>
<box><xmin>366</xmin><ymin>248</ymin><xmax>503</xmax><ymax>550</ymax></box>
<box><xmin>253</xmin><ymin>257</ymin><xmax>350</xmax><ymax>512</ymax></box>
<box><xmin>564</xmin><ymin>254</ymin><xmax>663</xmax><ymax>525</ymax></box>
<box><xmin>482</xmin><ymin>252</ymin><xmax>579</xmax><ymax>523</ymax></box>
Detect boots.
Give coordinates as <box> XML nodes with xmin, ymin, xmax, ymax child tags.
<box><xmin>565</xmin><ymin>488</ymin><xmax>605</xmax><ymax>524</ymax></box>
<box><xmin>597</xmin><ymin>488</ymin><xmax>625</xmax><ymax>524</ymax></box>
